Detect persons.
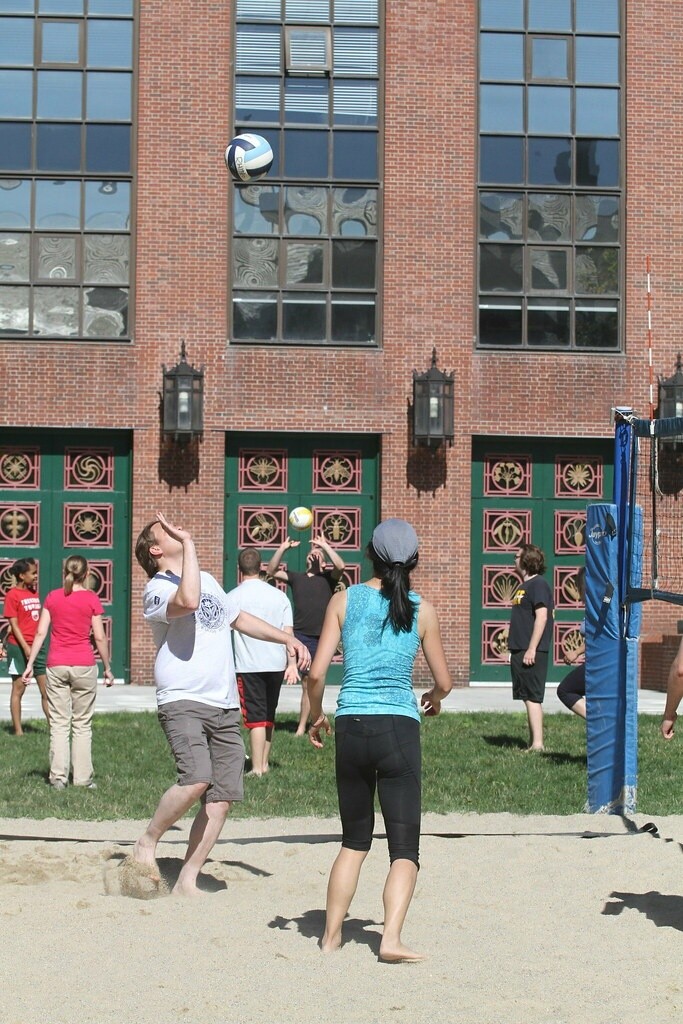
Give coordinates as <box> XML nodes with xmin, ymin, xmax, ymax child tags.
<box><xmin>660</xmin><ymin>636</ymin><xmax>683</xmax><ymax>739</ymax></box>
<box><xmin>508</xmin><ymin>544</ymin><xmax>554</xmax><ymax>754</ymax></box>
<box><xmin>21</xmin><ymin>555</ymin><xmax>114</xmax><ymax>790</ymax></box>
<box><xmin>307</xmin><ymin>519</ymin><xmax>453</xmax><ymax>961</ymax></box>
<box><xmin>2</xmin><ymin>558</ymin><xmax>50</xmax><ymax>735</ymax></box>
<box><xmin>227</xmin><ymin>533</ymin><xmax>345</xmax><ymax>778</ymax></box>
<box><xmin>557</xmin><ymin>567</ymin><xmax>587</xmax><ymax>720</ymax></box>
<box><xmin>132</xmin><ymin>511</ymin><xmax>312</xmax><ymax>896</ymax></box>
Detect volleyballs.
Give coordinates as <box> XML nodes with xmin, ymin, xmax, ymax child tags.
<box><xmin>223</xmin><ymin>132</ymin><xmax>274</xmax><ymax>182</ymax></box>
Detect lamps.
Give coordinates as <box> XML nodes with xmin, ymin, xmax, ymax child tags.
<box><xmin>406</xmin><ymin>347</ymin><xmax>455</xmax><ymax>449</ymax></box>
<box><xmin>156</xmin><ymin>341</ymin><xmax>206</xmax><ymax>443</ymax></box>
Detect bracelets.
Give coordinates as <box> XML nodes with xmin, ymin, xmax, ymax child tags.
<box><xmin>310</xmin><ymin>714</ymin><xmax>325</xmax><ymax>727</ymax></box>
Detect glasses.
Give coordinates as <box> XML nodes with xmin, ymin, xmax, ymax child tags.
<box><xmin>515</xmin><ymin>555</ymin><xmax>522</xmax><ymax>560</ymax></box>
